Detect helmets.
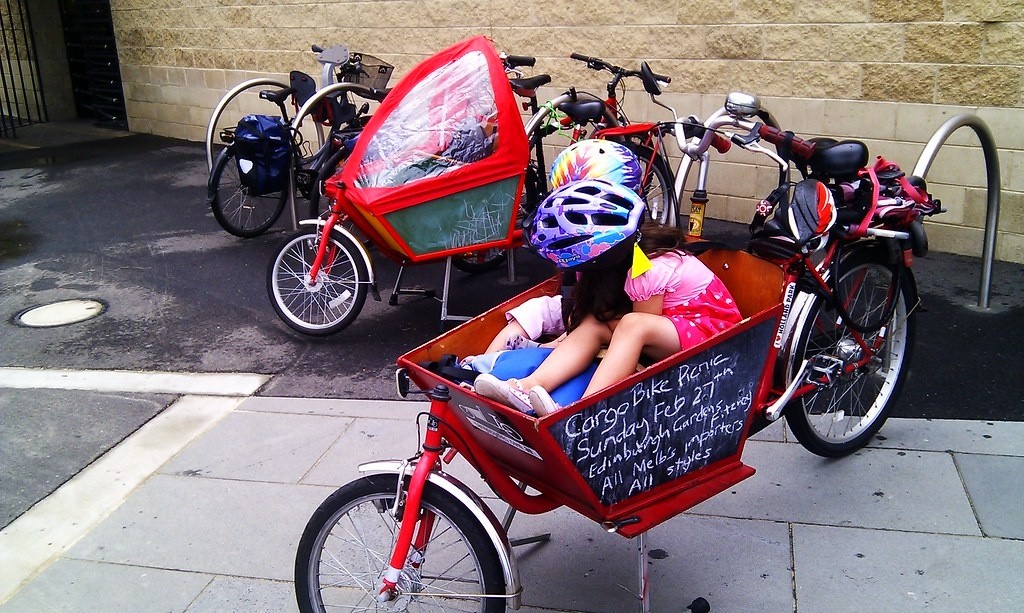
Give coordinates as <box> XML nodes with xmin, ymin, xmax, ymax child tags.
<box><xmin>521</xmin><ymin>179</ymin><xmax>645</xmax><ymax>269</ymax></box>
<box><xmin>549</xmin><ymin>139</ymin><xmax>644</xmax><ymax>198</ymax></box>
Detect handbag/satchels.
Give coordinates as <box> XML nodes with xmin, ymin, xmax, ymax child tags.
<box><xmin>234</xmin><ymin>114</ymin><xmax>290</xmax><ymax>196</ymax></box>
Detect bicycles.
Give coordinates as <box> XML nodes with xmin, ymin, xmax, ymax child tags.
<box><xmin>204</xmin><ymin>42</ymin><xmax>537</xmax><ymax>254</ymax></box>
<box><xmin>445</xmin><ymin>53</ymin><xmax>671</xmax><ymax>272</ymax></box>
<box><xmin>295</xmin><ymin>64</ymin><xmax>928</xmax><ymax>613</ymax></box>
<box><xmin>267</xmin><ymin>36</ymin><xmax>673</xmax><ymax>339</ymax></box>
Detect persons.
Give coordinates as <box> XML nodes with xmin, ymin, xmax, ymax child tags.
<box><xmin>474</xmin><ymin>139</ymin><xmax>743</xmax><ymax>418</ymax></box>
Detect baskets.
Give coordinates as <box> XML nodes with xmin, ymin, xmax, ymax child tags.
<box><xmin>342</xmin><ymin>52</ymin><xmax>394</xmax><ymax>90</ymax></box>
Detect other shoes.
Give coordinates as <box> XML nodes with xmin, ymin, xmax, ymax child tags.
<box><xmin>529</xmin><ymin>385</ymin><xmax>561</xmax><ymax>417</ymax></box>
<box><xmin>474</xmin><ymin>373</ymin><xmax>533</xmax><ymax>413</ymax></box>
